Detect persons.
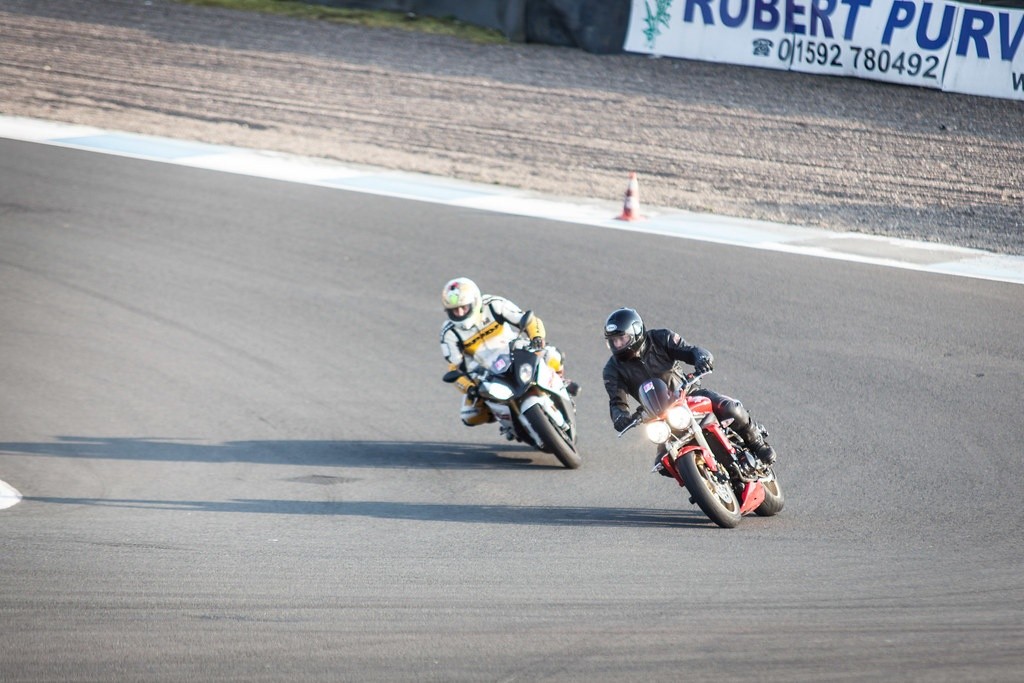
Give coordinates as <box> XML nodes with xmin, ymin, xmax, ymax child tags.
<box><xmin>602</xmin><ymin>307</ymin><xmax>776</xmax><ymax>505</ymax></box>
<box><xmin>440</xmin><ymin>278</ymin><xmax>580</xmax><ymax>441</ymax></box>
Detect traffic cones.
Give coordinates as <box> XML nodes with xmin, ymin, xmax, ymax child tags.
<box><xmin>612</xmin><ymin>173</ymin><xmax>647</xmax><ymax>223</ymax></box>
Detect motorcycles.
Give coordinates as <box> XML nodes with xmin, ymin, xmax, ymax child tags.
<box><xmin>618</xmin><ymin>371</ymin><xmax>785</xmax><ymax>529</ymax></box>
<box><xmin>442</xmin><ymin>310</ymin><xmax>582</xmax><ymax>470</ymax></box>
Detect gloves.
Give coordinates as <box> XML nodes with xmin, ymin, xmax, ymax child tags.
<box><xmin>468</xmin><ymin>386</ymin><xmax>481</xmax><ymax>402</ymax></box>
<box><xmin>611</xmin><ymin>407</ymin><xmax>631</xmax><ymax>432</ymax></box>
<box><xmin>529</xmin><ymin>337</ymin><xmax>545</xmax><ymax>352</ymax></box>
<box><xmin>695</xmin><ymin>356</ymin><xmax>714</xmax><ymax>379</ymax></box>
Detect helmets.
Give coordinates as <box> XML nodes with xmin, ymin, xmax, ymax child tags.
<box><xmin>604</xmin><ymin>307</ymin><xmax>647</xmax><ymax>363</ymax></box>
<box><xmin>441</xmin><ymin>277</ymin><xmax>482</xmax><ymax>330</ymax></box>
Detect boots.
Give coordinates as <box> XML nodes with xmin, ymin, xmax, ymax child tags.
<box><xmin>505</xmin><ymin>432</ymin><xmax>515</xmax><ymax>441</ymax></box>
<box><xmin>556</xmin><ymin>368</ymin><xmax>580</xmax><ymax>396</ymax></box>
<box><xmin>736</xmin><ymin>417</ymin><xmax>777</xmax><ymax>464</ymax></box>
